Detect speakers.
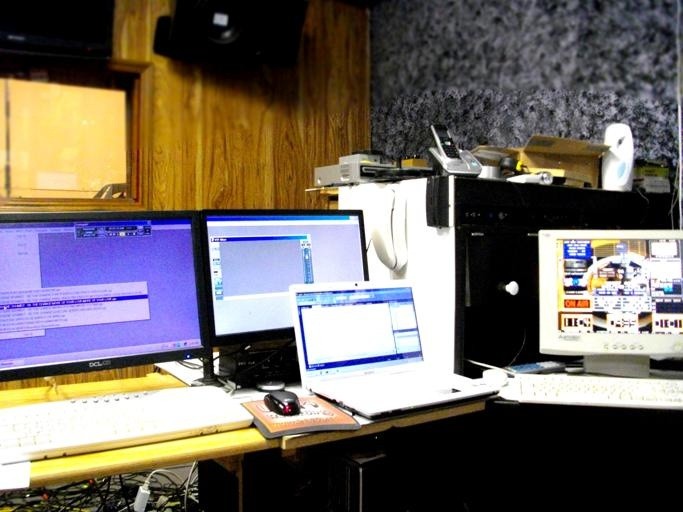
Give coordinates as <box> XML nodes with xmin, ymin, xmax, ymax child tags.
<box><xmin>0</xmin><ymin>0</ymin><xmax>116</xmax><ymax>76</ymax></box>
<box><xmin>164</xmin><ymin>0</ymin><xmax>311</xmax><ymax>75</ymax></box>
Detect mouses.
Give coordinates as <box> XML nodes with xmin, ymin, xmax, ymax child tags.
<box><xmin>484</xmin><ymin>364</ymin><xmax>512</xmax><ymax>390</ymax></box>
<box><xmin>264</xmin><ymin>389</ymin><xmax>303</xmax><ymax>414</ymax></box>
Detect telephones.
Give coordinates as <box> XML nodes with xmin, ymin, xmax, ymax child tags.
<box><xmin>429</xmin><ymin>124</ymin><xmax>482</xmax><ymax>177</ymax></box>
<box><xmin>371</xmin><ymin>182</ymin><xmax>408</xmax><ymax>273</ymax></box>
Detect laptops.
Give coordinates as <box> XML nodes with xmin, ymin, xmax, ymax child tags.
<box><xmin>291</xmin><ymin>278</ymin><xmax>499</xmax><ymax>419</ymax></box>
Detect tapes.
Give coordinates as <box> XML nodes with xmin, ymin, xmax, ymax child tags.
<box><xmin>477</xmin><ymin>166</ymin><xmax>501</xmax><ymax>178</ymax></box>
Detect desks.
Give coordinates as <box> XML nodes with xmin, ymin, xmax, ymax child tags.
<box><xmin>487</xmin><ymin>351</ymin><xmax>682</xmax><ymax>416</ymax></box>
<box><xmin>1</xmin><ymin>350</ymin><xmax>487</xmax><ymax>507</ymax></box>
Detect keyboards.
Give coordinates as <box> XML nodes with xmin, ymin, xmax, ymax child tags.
<box><xmin>515</xmin><ymin>372</ymin><xmax>683</xmax><ymax>412</ymax></box>
<box><xmin>0</xmin><ymin>383</ymin><xmax>255</xmax><ymax>466</ymax></box>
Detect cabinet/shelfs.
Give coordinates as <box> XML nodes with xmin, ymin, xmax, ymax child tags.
<box><xmin>336</xmin><ymin>175</ymin><xmax>680</xmax><ymax>389</ymax></box>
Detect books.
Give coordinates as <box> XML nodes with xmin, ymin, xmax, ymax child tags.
<box><xmin>239</xmin><ymin>394</ymin><xmax>361</xmax><ymax>439</ymax></box>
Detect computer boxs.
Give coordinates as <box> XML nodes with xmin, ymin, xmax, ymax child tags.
<box><xmin>339</xmin><ymin>174</ymin><xmax>545</xmax><ymax>376</ymax></box>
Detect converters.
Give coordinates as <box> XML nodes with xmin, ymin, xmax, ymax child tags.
<box><xmin>133</xmin><ymin>485</ymin><xmax>150</xmax><ymax>511</ymax></box>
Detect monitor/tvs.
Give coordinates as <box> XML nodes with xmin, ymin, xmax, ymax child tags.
<box><xmin>0</xmin><ymin>208</ymin><xmax>213</xmax><ymax>384</ymax></box>
<box><xmin>200</xmin><ymin>208</ymin><xmax>369</xmax><ymax>347</ymax></box>
<box><xmin>536</xmin><ymin>227</ymin><xmax>682</xmax><ymax>378</ymax></box>
